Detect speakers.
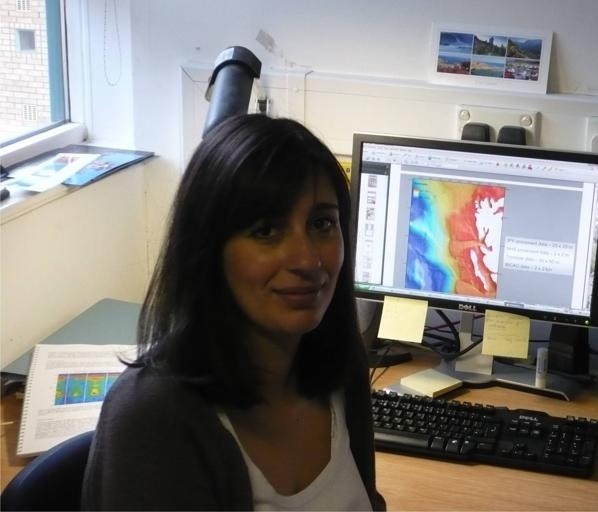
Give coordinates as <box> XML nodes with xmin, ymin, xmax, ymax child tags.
<box><xmin>352</xmin><ymin>297</ymin><xmax>414</xmax><ymax>368</ymax></box>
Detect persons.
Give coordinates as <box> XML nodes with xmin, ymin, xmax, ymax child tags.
<box><xmin>75</xmin><ymin>114</ymin><xmax>391</xmax><ymax>510</ymax></box>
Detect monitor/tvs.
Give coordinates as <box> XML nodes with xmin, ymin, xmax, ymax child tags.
<box><xmin>348</xmin><ymin>133</ymin><xmax>598</xmax><ymax>402</ymax></box>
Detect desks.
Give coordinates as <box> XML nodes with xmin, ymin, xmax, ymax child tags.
<box><xmin>1</xmin><ymin>341</ymin><xmax>598</xmax><ymax>512</ymax></box>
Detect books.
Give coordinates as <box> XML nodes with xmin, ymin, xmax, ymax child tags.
<box><xmin>4</xmin><ymin>145</ymin><xmax>156</xmax><ymax>190</ymax></box>
<box><xmin>1</xmin><ymin>298</ymin><xmax>160</xmax><ymax>459</ymax></box>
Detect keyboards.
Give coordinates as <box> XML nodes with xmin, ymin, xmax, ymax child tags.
<box><xmin>370</xmin><ymin>388</ymin><xmax>598</xmax><ymax>480</ymax></box>
